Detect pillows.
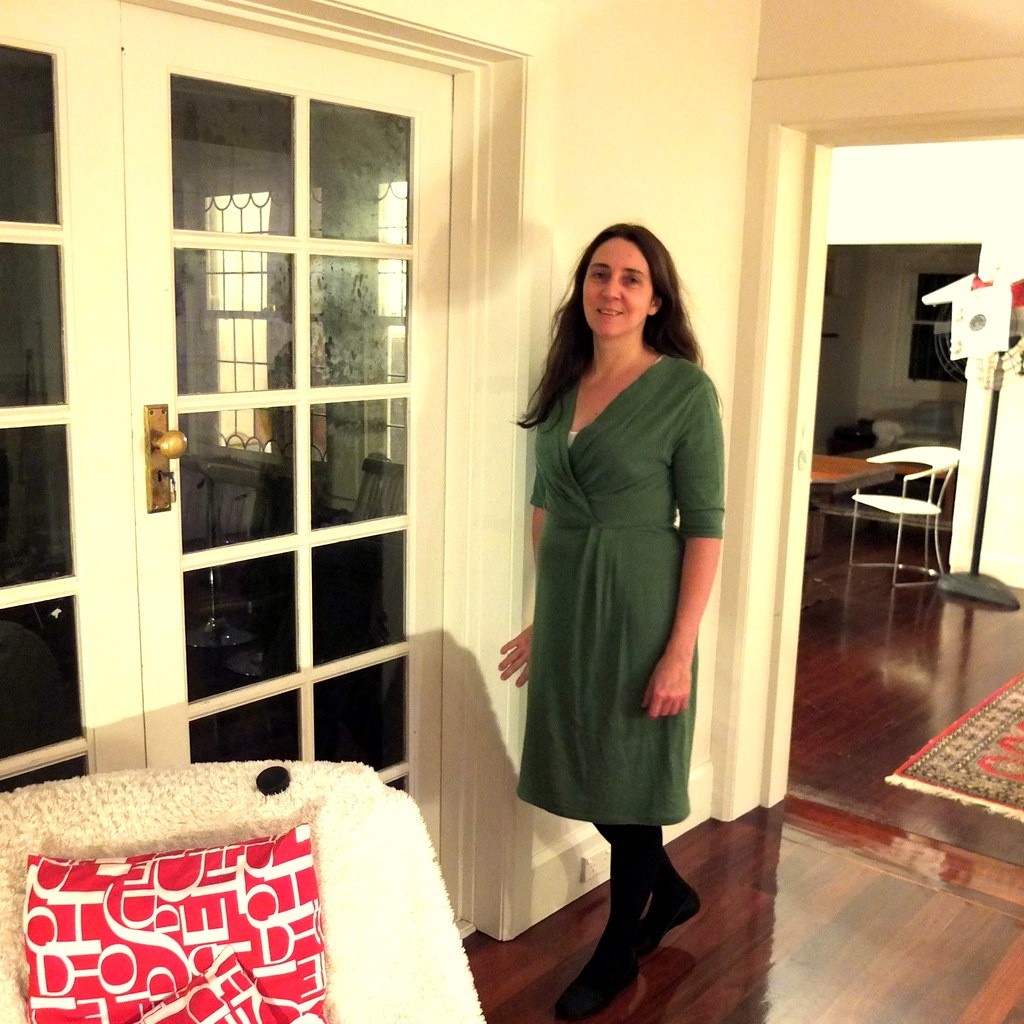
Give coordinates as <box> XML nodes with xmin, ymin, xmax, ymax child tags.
<box><xmin>22</xmin><ymin>822</ymin><xmax>328</xmax><ymax>1024</ymax></box>
<box><xmin>133</xmin><ymin>944</ymin><xmax>280</xmax><ymax>1024</ymax></box>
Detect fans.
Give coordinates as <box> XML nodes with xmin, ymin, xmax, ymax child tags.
<box><xmin>933</xmin><ymin>275</ymin><xmax>1024</xmax><ymax>610</ymax></box>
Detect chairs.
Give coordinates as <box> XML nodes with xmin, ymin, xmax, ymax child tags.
<box><xmin>848</xmin><ymin>445</ymin><xmax>960</xmax><ymax>587</ymax></box>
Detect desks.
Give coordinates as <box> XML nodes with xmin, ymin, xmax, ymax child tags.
<box><xmin>844</xmin><ymin>448</ymin><xmax>947</xmax><ymax>480</ymax></box>
<box><xmin>800</xmin><ymin>455</ymin><xmax>897</xmax><ymax>611</ymax></box>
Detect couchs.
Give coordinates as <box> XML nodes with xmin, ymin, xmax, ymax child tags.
<box><xmin>872</xmin><ymin>400</ymin><xmax>966</xmax><ymax>450</ymax></box>
<box><xmin>0</xmin><ymin>760</ymin><xmax>488</xmax><ymax>1024</ymax></box>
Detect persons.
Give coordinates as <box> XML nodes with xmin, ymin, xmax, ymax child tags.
<box><xmin>499</xmin><ymin>222</ymin><xmax>725</xmax><ymax>1017</ymax></box>
